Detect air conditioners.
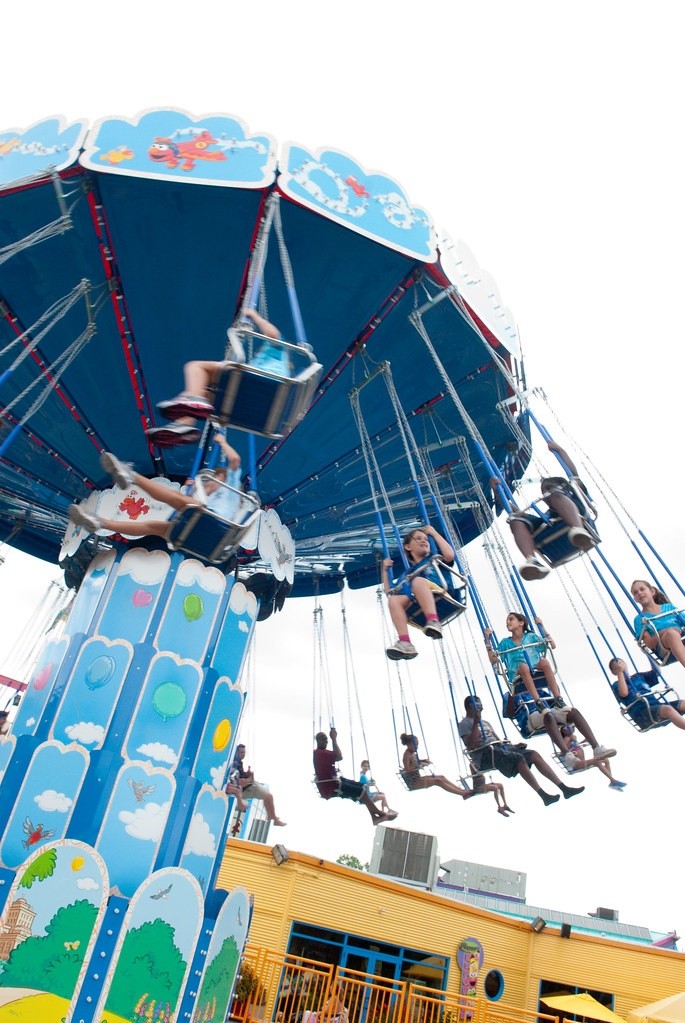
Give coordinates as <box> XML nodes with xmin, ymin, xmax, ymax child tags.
<box><xmin>597</xmin><ymin>907</ymin><xmax>619</xmax><ymax>921</ymax></box>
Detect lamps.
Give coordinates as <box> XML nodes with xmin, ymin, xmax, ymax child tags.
<box><xmin>271</xmin><ymin>844</ymin><xmax>288</xmax><ymax>865</ymax></box>
<box><xmin>531</xmin><ymin>916</ymin><xmax>546</xmax><ymax>934</ymax></box>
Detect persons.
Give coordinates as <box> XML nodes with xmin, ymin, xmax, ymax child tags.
<box><xmin>360</xmin><ymin>761</ymin><xmax>395</xmax><ymax>813</ymax></box>
<box><xmin>400</xmin><ymin>734</ymin><xmax>477</xmax><ymax>800</ymax></box>
<box><xmin>488</xmin><ymin>441</ymin><xmax>596</xmax><ymax>581</ymax></box>
<box><xmin>631</xmin><ymin>580</ymin><xmax>685</xmax><ymax>668</ymax></box>
<box><xmin>458</xmin><ymin>695</ymin><xmax>585</xmax><ymax>806</ymax></box>
<box><xmin>225</xmin><ymin>744</ymin><xmax>286</xmax><ymax>826</ymax></box>
<box><xmin>0</xmin><ymin>711</ymin><xmax>11</xmax><ymax>737</ymax></box>
<box><xmin>68</xmin><ymin>433</ymin><xmax>241</xmax><ymax>542</ymax></box>
<box><xmin>143</xmin><ymin>310</ymin><xmax>286</xmax><ymax>444</ymax></box>
<box><xmin>484</xmin><ymin>613</ymin><xmax>570</xmax><ymax>715</ymax></box>
<box><xmin>503</xmin><ymin>688</ymin><xmax>617</xmax><ymax>765</ymax></box>
<box><xmin>561</xmin><ymin>726</ymin><xmax>627</xmax><ymax>792</ymax></box>
<box><xmin>609</xmin><ymin>657</ymin><xmax>685</xmax><ymax>730</ymax></box>
<box><xmin>380</xmin><ymin>525</ymin><xmax>455</xmax><ymax>660</ymax></box>
<box><xmin>314</xmin><ymin>727</ymin><xmax>398</xmax><ymax>824</ymax></box>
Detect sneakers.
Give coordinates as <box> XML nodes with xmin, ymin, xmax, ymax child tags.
<box><xmin>156</xmin><ymin>394</ymin><xmax>213</xmax><ymax>420</ymax></box>
<box><xmin>387</xmin><ymin>640</ymin><xmax>418</xmax><ymax>660</ymax></box>
<box><xmin>563</xmin><ymin>750</ymin><xmax>581</xmax><ymax>767</ymax></box>
<box><xmin>568</xmin><ymin>528</ymin><xmax>596</xmax><ymax>552</ymax></box>
<box><xmin>593</xmin><ymin>745</ymin><xmax>616</xmax><ymax>759</ymax></box>
<box><xmin>146</xmin><ymin>426</ymin><xmax>200</xmax><ymax>448</ymax></box>
<box><xmin>519</xmin><ymin>557</ymin><xmax>549</xmax><ymax>581</ymax></box>
<box><xmin>423</xmin><ymin>620</ymin><xmax>443</xmax><ymax>639</ymax></box>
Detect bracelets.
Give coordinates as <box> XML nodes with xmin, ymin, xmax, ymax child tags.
<box><xmin>546</xmin><ymin>633</ymin><xmax>550</xmax><ymax>637</ymax></box>
<box><xmin>485</xmin><ymin>644</ymin><xmax>492</xmax><ymax>651</ymax></box>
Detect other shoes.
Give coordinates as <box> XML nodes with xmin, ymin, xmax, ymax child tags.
<box><xmin>69</xmin><ymin>505</ymin><xmax>103</xmax><ymax>533</ymax></box>
<box><xmin>564</xmin><ymin>787</ymin><xmax>585</xmax><ymax>798</ymax></box>
<box><xmin>274</xmin><ymin>820</ymin><xmax>285</xmax><ymax>826</ymax></box>
<box><xmin>237</xmin><ymin>804</ymin><xmax>248</xmax><ymax>810</ymax></box>
<box><xmin>463</xmin><ymin>789</ymin><xmax>477</xmax><ymax>800</ymax></box>
<box><xmin>504</xmin><ymin>806</ymin><xmax>515</xmax><ymax>814</ymax></box>
<box><xmin>373</xmin><ymin>810</ymin><xmax>397</xmax><ymax>825</ymax></box>
<box><xmin>101</xmin><ymin>453</ymin><xmax>133</xmax><ymax>490</ymax></box>
<box><xmin>609</xmin><ymin>780</ymin><xmax>627</xmax><ymax>792</ymax></box>
<box><xmin>498</xmin><ymin>807</ymin><xmax>510</xmax><ymax>817</ymax></box>
<box><xmin>544</xmin><ymin>794</ymin><xmax>560</xmax><ymax>806</ymax></box>
